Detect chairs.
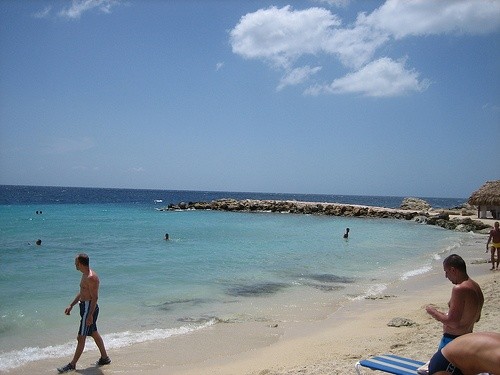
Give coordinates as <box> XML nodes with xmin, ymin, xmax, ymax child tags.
<box><xmin>355</xmin><ymin>354</ymin><xmax>430</xmax><ymax>375</ymax></box>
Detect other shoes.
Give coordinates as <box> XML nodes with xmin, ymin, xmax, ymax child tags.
<box><xmin>98</xmin><ymin>356</ymin><xmax>111</xmax><ymax>365</ymax></box>
<box><xmin>57</xmin><ymin>363</ymin><xmax>75</xmax><ymax>373</ymax></box>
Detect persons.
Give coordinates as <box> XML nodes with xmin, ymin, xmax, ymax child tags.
<box><xmin>56</xmin><ymin>253</ymin><xmax>111</xmax><ymax>373</ymax></box>
<box><xmin>486</xmin><ymin>222</ymin><xmax>500</xmax><ymax>271</ymax></box>
<box><xmin>36</xmin><ymin>239</ymin><xmax>42</xmax><ymax>245</ymax></box>
<box><xmin>344</xmin><ymin>228</ymin><xmax>349</xmax><ymax>238</ymax></box>
<box><xmin>426</xmin><ymin>255</ymin><xmax>484</xmax><ymax>350</ymax></box>
<box><xmin>163</xmin><ymin>234</ymin><xmax>170</xmax><ymax>240</ymax></box>
<box><xmin>428</xmin><ymin>331</ymin><xmax>500</xmax><ymax>375</ymax></box>
